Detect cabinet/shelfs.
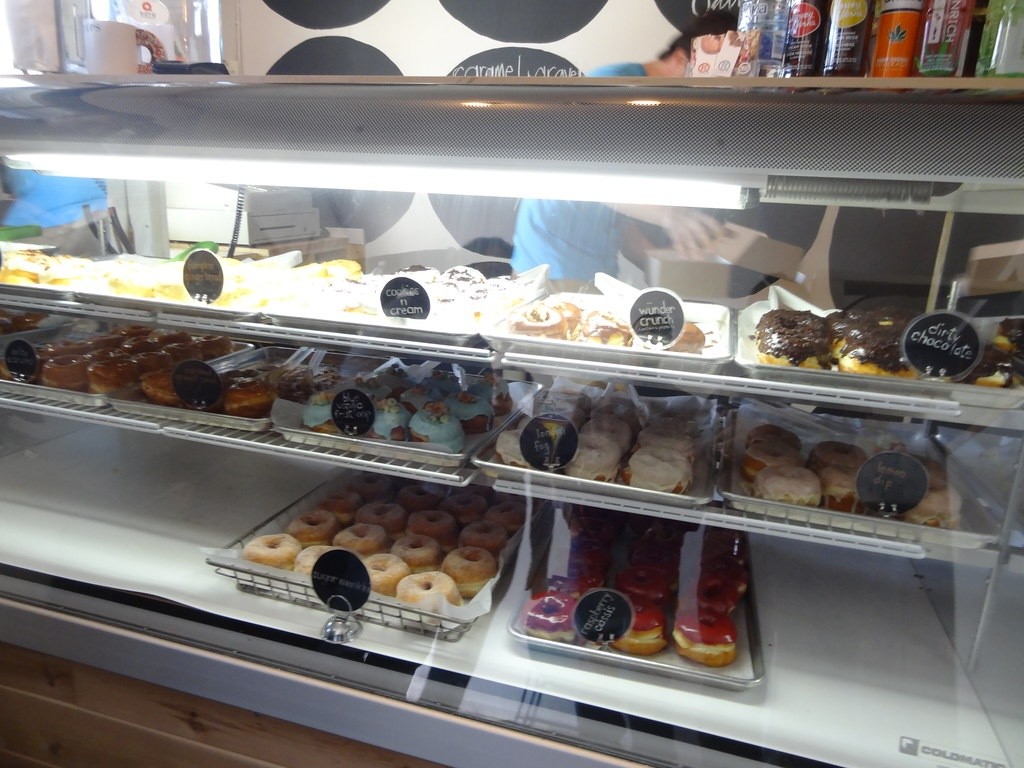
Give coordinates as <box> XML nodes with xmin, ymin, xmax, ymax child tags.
<box><xmin>1</xmin><ymin>257</ymin><xmax>1024</xmax><ymax>768</ymax></box>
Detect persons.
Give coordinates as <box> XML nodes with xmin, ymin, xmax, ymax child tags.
<box><xmin>0</xmin><ymin>165</ymin><xmax>110</xmax><ymax>256</ymax></box>
<box><xmin>508</xmin><ymin>10</ymin><xmax>740</xmax><ymax>293</ymax></box>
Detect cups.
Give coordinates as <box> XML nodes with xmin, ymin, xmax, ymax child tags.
<box><xmin>83</xmin><ymin>19</ymin><xmax>165</xmax><ymax>75</ymax></box>
<box><xmin>137</xmin><ymin>23</ymin><xmax>176</xmax><ymax>63</ymax></box>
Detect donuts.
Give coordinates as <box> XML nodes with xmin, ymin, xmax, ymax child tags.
<box><xmin>0</xmin><ymin>250</ymin><xmax>706</xmax><ymax>356</ymax></box>
<box><xmin>746</xmin><ymin>300</ymin><xmax>1024</xmax><ymax>393</ymax></box>
<box><xmin>244</xmin><ymin>468</ymin><xmax>538</xmax><ymax>620</ymax></box>
<box><xmin>0</xmin><ymin>307</ymin><xmax>707</xmax><ymax>496</ymax></box>
<box><xmin>519</xmin><ymin>505</ymin><xmax>749</xmax><ymax>666</ymax></box>
<box><xmin>739</xmin><ymin>423</ymin><xmax>961</xmax><ymax>529</ymax></box>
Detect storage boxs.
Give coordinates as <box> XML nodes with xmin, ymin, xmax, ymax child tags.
<box><xmin>649</xmin><ymin>225</ymin><xmax>807</xmax><ymax>299</ymax></box>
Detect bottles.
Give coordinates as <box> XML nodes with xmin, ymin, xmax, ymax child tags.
<box><xmin>737</xmin><ymin>0</ymin><xmax>789</xmax><ymax>77</ymax></box>
